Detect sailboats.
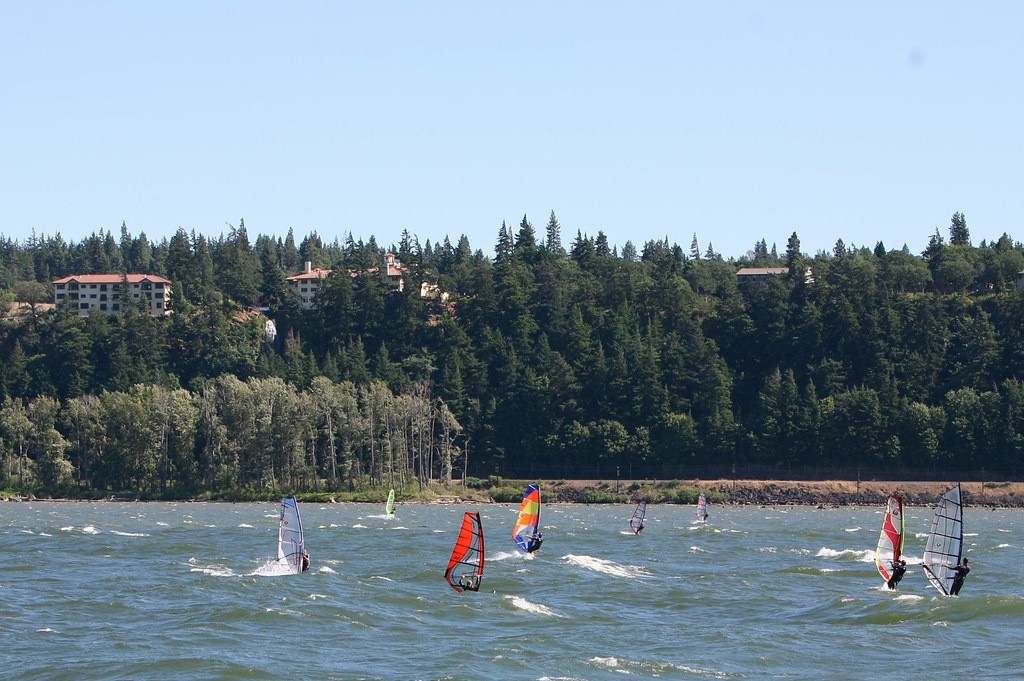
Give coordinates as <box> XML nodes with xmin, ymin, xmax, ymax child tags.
<box><xmin>875</xmin><ymin>493</ymin><xmax>905</xmax><ymax>592</ymax></box>
<box><xmin>385</xmin><ymin>488</ymin><xmax>396</xmax><ymax>515</ymax></box>
<box><xmin>695</xmin><ymin>490</ymin><xmax>707</xmax><ymax>521</ymax></box>
<box><xmin>443</xmin><ymin>511</ymin><xmax>485</xmax><ymax>594</ymax></box>
<box><xmin>920</xmin><ymin>481</ymin><xmax>963</xmax><ymax>599</ymax></box>
<box><xmin>630</xmin><ymin>499</ymin><xmax>646</xmax><ymax>536</ymax></box>
<box><xmin>278</xmin><ymin>494</ymin><xmax>304</xmax><ymax>575</ymax></box>
<box><xmin>511</xmin><ymin>484</ymin><xmax>541</xmax><ymax>554</ymax></box>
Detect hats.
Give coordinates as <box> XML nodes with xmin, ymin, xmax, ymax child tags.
<box><xmin>539</xmin><ymin>532</ymin><xmax>543</xmax><ymax>538</ymax></box>
<box><xmin>901</xmin><ymin>559</ymin><xmax>907</xmax><ymax>566</ymax></box>
<box><xmin>963</xmin><ymin>558</ymin><xmax>969</xmax><ymax>563</ymax></box>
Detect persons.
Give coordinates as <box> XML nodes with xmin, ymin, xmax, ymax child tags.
<box><xmin>304</xmin><ymin>554</ymin><xmax>310</xmax><ymax>570</ymax></box>
<box><xmin>459</xmin><ymin>575</ymin><xmax>477</xmax><ymax>591</ymax></box>
<box><xmin>528</xmin><ymin>533</ymin><xmax>544</xmax><ymax>553</ymax></box>
<box><xmin>636</xmin><ymin>522</ymin><xmax>644</xmax><ymax>533</ymax></box>
<box><xmin>889</xmin><ymin>559</ymin><xmax>906</xmax><ymax>590</ymax></box>
<box><xmin>390</xmin><ymin>506</ymin><xmax>396</xmax><ymax>514</ymax></box>
<box><xmin>704</xmin><ymin>512</ymin><xmax>709</xmax><ymax>520</ymax></box>
<box><xmin>947</xmin><ymin>557</ymin><xmax>971</xmax><ymax>596</ymax></box>
